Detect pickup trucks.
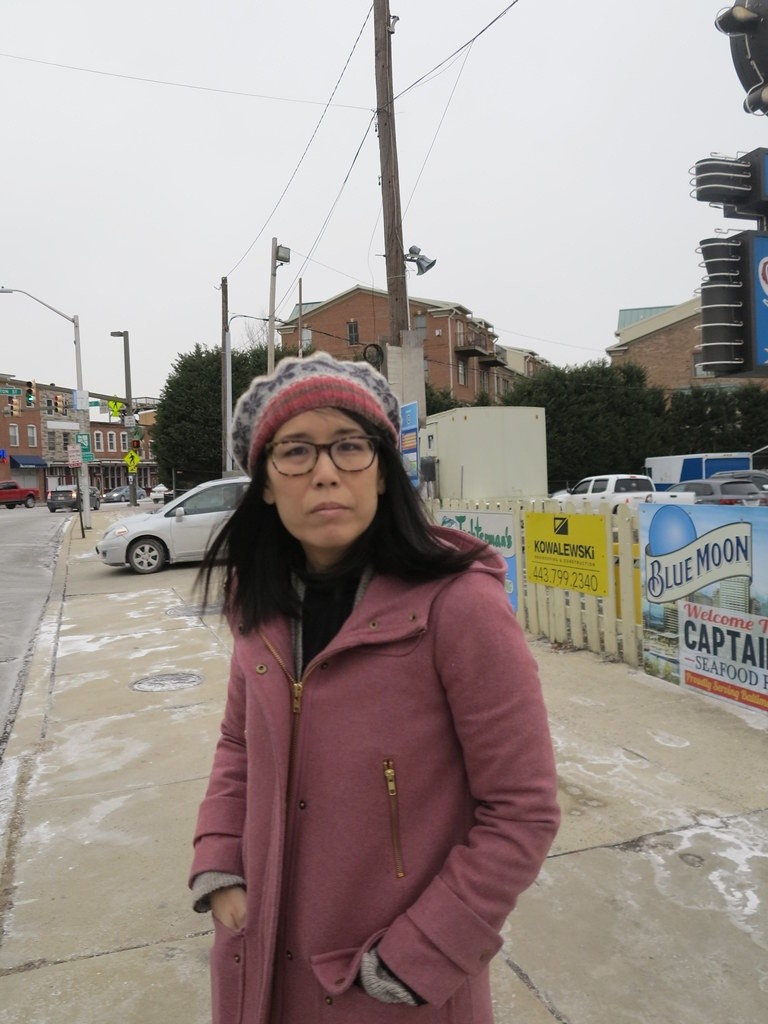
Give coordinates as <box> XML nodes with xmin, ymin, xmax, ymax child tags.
<box><xmin>549</xmin><ymin>473</ymin><xmax>696</xmax><ymax>514</ymax></box>
<box><xmin>0</xmin><ymin>480</ymin><xmax>40</xmax><ymax>509</ymax></box>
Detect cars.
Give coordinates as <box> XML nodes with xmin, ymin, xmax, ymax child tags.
<box><xmin>47</xmin><ymin>485</ymin><xmax>100</xmax><ymax>513</ymax></box>
<box><xmin>148</xmin><ymin>483</ymin><xmax>174</xmax><ymax>504</ymax></box>
<box><xmin>102</xmin><ymin>484</ymin><xmax>146</xmax><ymax>504</ymax></box>
<box><xmin>90</xmin><ymin>475</ymin><xmax>254</xmax><ymax>577</ymax></box>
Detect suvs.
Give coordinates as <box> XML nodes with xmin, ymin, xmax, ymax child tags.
<box><xmin>706</xmin><ymin>469</ymin><xmax>768</xmax><ymax>496</ymax></box>
<box><xmin>664</xmin><ymin>474</ymin><xmax>768</xmax><ymax>506</ymax></box>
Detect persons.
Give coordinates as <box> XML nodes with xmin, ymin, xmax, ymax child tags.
<box><xmin>188</xmin><ymin>352</ymin><xmax>561</xmax><ymax>1024</ymax></box>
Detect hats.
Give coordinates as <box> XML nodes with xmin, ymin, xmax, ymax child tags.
<box><xmin>229</xmin><ymin>350</ymin><xmax>402</xmax><ymax>478</ymax></box>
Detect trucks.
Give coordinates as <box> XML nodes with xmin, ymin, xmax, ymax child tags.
<box><xmin>641</xmin><ymin>452</ymin><xmax>753</xmax><ymax>492</ymax></box>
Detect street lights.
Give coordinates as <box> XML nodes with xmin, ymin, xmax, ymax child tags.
<box><xmin>263</xmin><ymin>236</ymin><xmax>292</xmax><ymax>376</ymax></box>
<box><xmin>111</xmin><ymin>331</ymin><xmax>140</xmax><ymax>506</ymax></box>
<box><xmin>0</xmin><ymin>286</ymin><xmax>94</xmax><ymax>530</ymax></box>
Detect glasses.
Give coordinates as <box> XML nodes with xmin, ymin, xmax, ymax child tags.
<box><xmin>265</xmin><ymin>434</ymin><xmax>381</xmax><ymax>476</ymax></box>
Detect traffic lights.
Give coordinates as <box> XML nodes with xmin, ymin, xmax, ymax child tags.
<box><xmin>25</xmin><ymin>381</ymin><xmax>37</xmax><ymax>403</ymax></box>
<box><xmin>131</xmin><ymin>439</ymin><xmax>141</xmax><ymax>448</ymax></box>
<box><xmin>25</xmin><ymin>389</ymin><xmax>35</xmax><ymax>409</ymax></box>
<box><xmin>10</xmin><ymin>398</ymin><xmax>21</xmax><ymax>418</ymax></box>
<box><xmin>53</xmin><ymin>395</ymin><xmax>64</xmax><ymax>414</ymax></box>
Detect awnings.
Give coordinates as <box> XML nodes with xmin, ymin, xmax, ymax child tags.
<box><xmin>9</xmin><ymin>454</ymin><xmax>48</xmax><ymax>469</ymax></box>
